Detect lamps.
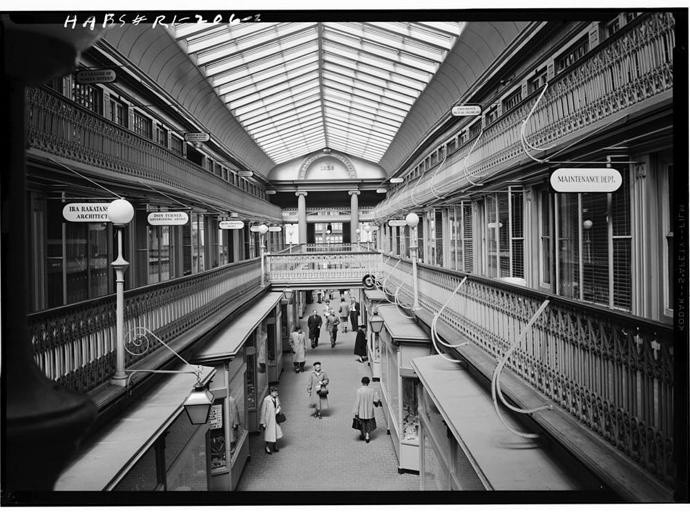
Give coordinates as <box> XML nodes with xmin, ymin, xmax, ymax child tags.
<box><xmin>406</xmin><ymin>213</ymin><xmax>420</xmax><ymax>309</ymax></box>
<box><xmin>370</xmin><ymin>301</ymin><xmax>415</xmax><ymax>334</ymax></box>
<box><xmin>119</xmin><ymin>323</ymin><xmax>213</xmax><ymax>426</ymax></box>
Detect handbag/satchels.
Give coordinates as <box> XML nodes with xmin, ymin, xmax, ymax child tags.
<box><xmin>276</xmin><ymin>412</ymin><xmax>286</xmax><ymax>424</ymax></box>
<box><xmin>317</xmin><ymin>389</ymin><xmax>329</xmax><ymax>397</ymax></box>
<box><xmin>352</xmin><ymin>418</ymin><xmax>363</xmax><ymax>430</ymax></box>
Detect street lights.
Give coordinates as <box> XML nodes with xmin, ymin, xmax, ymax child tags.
<box><xmin>288</xmin><ymin>230</ymin><xmax>293</xmax><ymax>248</ymax></box>
<box><xmin>406</xmin><ymin>213</ymin><xmax>422</xmax><ymax>311</ymax></box>
<box><xmin>355</xmin><ymin>229</ymin><xmax>360</xmax><ymax>246</ymax></box>
<box><xmin>364</xmin><ymin>226</ymin><xmax>371</xmax><ymax>251</ymax></box>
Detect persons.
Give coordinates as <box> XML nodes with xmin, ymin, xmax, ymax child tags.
<box><xmin>323</xmin><ymin>299</ymin><xmax>333</xmax><ymax>331</ymax></box>
<box><xmin>326</xmin><ymin>310</ymin><xmax>340</xmax><ymax>349</ymax></box>
<box><xmin>349</xmin><ymin>297</ymin><xmax>360</xmax><ymax>331</ymax></box>
<box><xmin>259</xmin><ymin>385</ymin><xmax>283</xmax><ymax>454</ymax></box>
<box><xmin>288</xmin><ymin>327</ymin><xmax>308</xmax><ymax>373</ymax></box>
<box><xmin>316</xmin><ymin>289</ymin><xmax>344</xmax><ymax>304</ymax></box>
<box><xmin>305</xmin><ymin>362</ymin><xmax>329</xmax><ymax>419</ymax></box>
<box><xmin>354</xmin><ymin>325</ymin><xmax>367</xmax><ymax>363</ymax></box>
<box><xmin>338</xmin><ymin>298</ymin><xmax>350</xmax><ymax>333</ymax></box>
<box><xmin>308</xmin><ymin>309</ymin><xmax>322</xmax><ymax>348</ymax></box>
<box><xmin>352</xmin><ymin>377</ymin><xmax>376</xmax><ymax>443</ymax></box>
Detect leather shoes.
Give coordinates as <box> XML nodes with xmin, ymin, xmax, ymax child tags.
<box><xmin>273</xmin><ymin>444</ymin><xmax>279</xmax><ymax>452</ymax></box>
<box><xmin>265</xmin><ymin>446</ymin><xmax>272</xmax><ymax>455</ymax></box>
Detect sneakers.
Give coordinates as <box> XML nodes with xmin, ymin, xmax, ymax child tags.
<box><xmin>360</xmin><ymin>435</ymin><xmax>370</xmax><ymax>443</ymax></box>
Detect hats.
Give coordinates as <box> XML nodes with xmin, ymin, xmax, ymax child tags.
<box><xmin>294</xmin><ymin>326</ymin><xmax>300</xmax><ymax>331</ymax></box>
<box><xmin>361</xmin><ymin>325</ymin><xmax>366</xmax><ymax>328</ymax></box>
